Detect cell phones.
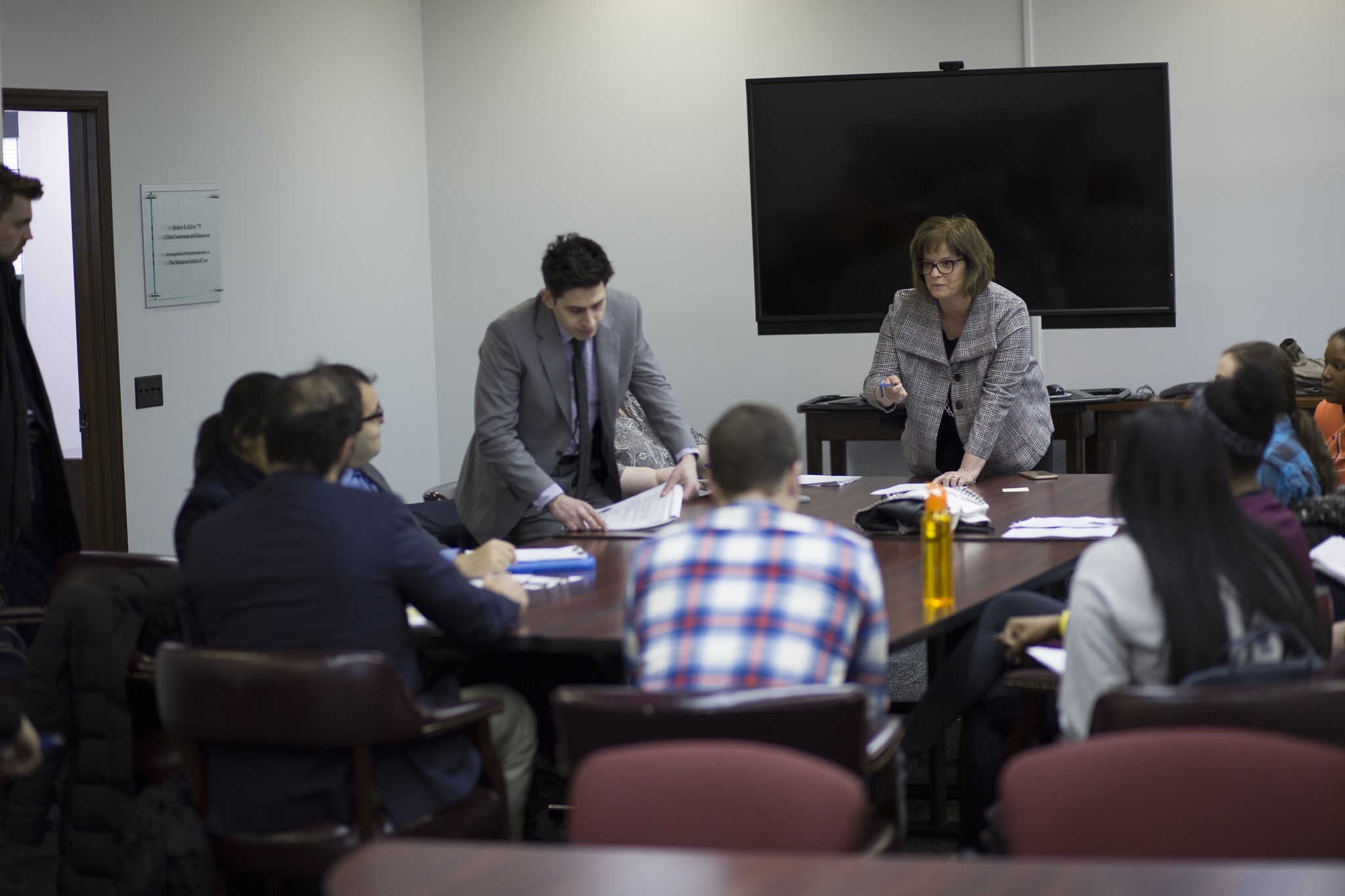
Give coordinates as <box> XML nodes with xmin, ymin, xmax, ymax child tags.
<box><xmin>1019</xmin><ymin>470</ymin><xmax>1057</xmax><ymax>480</ymax></box>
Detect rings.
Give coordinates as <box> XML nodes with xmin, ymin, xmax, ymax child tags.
<box><xmin>952</xmin><ymin>475</ymin><xmax>959</xmax><ymax>480</ymax></box>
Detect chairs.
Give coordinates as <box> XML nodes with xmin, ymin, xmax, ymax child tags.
<box><xmin>56</xmin><ymin>551</ymin><xmax>1345</xmax><ymax>861</ymax></box>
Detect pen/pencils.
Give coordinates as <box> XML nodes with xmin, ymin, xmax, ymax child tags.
<box><xmin>698</xmin><ymin>479</ymin><xmax>709</xmax><ymax>483</ymax></box>
<box><xmin>879</xmin><ymin>383</ymin><xmax>893</xmax><ymax>387</ymax></box>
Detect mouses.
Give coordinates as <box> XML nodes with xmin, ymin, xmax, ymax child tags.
<box><xmin>1046</xmin><ymin>384</ymin><xmax>1063</xmax><ymax>395</ymax></box>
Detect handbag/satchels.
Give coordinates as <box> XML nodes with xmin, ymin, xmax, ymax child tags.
<box><xmin>854</xmin><ymin>486</ymin><xmax>996</xmax><ymax>536</ymax></box>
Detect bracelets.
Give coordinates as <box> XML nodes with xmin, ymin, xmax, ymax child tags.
<box><xmin>959</xmin><ymin>468</ymin><xmax>976</xmax><ymax>485</ymax></box>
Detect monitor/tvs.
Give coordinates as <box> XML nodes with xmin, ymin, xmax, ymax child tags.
<box><xmin>1030</xmin><ymin>316</ymin><xmax>1042</xmax><ymax>367</ymax></box>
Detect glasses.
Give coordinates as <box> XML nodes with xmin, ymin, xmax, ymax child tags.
<box><xmin>919</xmin><ymin>257</ymin><xmax>963</xmax><ymax>275</ymax></box>
<box><xmin>364</xmin><ymin>404</ymin><xmax>384</xmax><ymax>424</ymax></box>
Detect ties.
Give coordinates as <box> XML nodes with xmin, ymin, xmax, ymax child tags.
<box><xmin>572</xmin><ymin>339</ymin><xmax>592</xmax><ymax>500</ymax></box>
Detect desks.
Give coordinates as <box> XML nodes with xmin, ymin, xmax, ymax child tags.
<box><xmin>797</xmin><ymin>389</ymin><xmax>1089</xmax><ymax>477</ymax></box>
<box><xmin>401</xmin><ymin>474</ymin><xmax>1125</xmax><ymax>660</ymax></box>
<box><xmin>1070</xmin><ymin>393</ymin><xmax>1324</xmax><ymax>472</ymax></box>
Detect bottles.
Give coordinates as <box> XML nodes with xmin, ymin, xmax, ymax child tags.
<box><xmin>920</xmin><ymin>489</ymin><xmax>956</xmax><ymax>608</ymax></box>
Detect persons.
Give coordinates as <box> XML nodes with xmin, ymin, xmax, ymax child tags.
<box><xmin>454</xmin><ymin>233</ymin><xmax>700</xmax><ymax>544</ymax></box>
<box><xmin>0</xmin><ymin>163</ymin><xmax>540</xmax><ymax>845</ymax></box>
<box><xmin>614</xmin><ymin>384</ymin><xmax>709</xmax><ymax>497</ymax></box>
<box><xmin>623</xmin><ymin>402</ymin><xmax>895</xmax><ymax>838</ymax></box>
<box><xmin>910</xmin><ymin>329</ymin><xmax>1345</xmax><ymax>825</ymax></box>
<box><xmin>864</xmin><ymin>216</ymin><xmax>1055</xmax><ymax>492</ymax></box>
